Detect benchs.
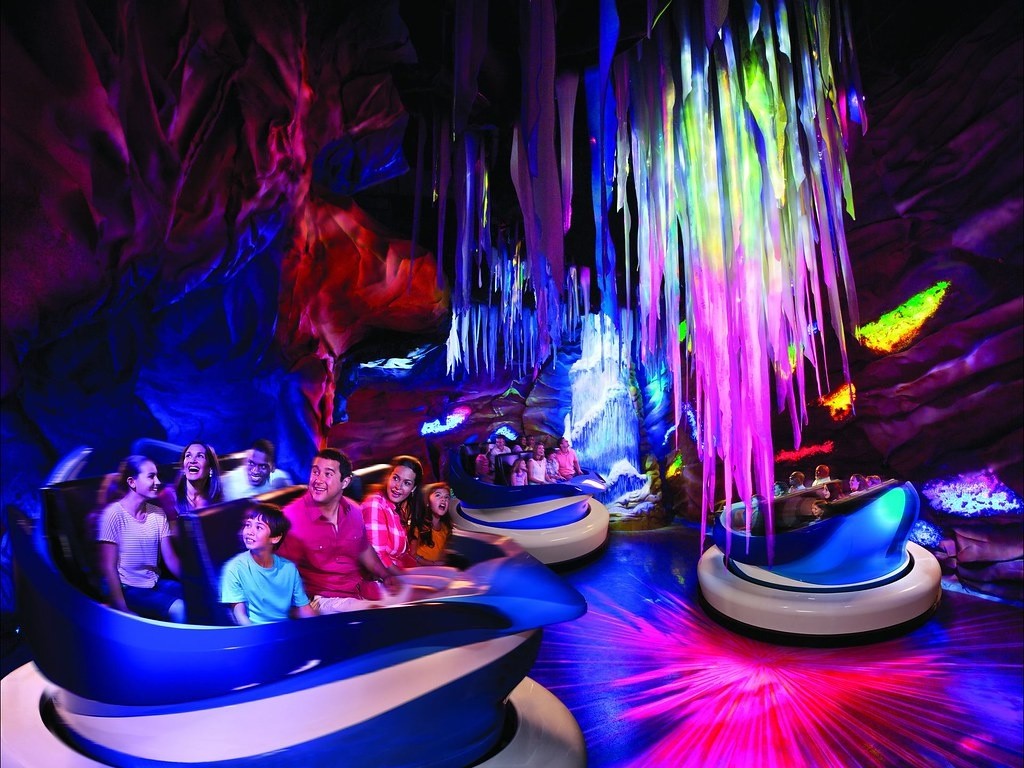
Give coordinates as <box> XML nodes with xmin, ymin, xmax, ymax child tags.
<box><xmin>448</xmin><ymin>440</ymin><xmax>594</xmax><ymax>528</ymax></box>
<box><xmin>5</xmin><ymin>450</ymin><xmax>591</xmax><ymax>768</ymax></box>
<box><xmin>705</xmin><ymin>476</ymin><xmax>921</xmax><ymax>590</ymax></box>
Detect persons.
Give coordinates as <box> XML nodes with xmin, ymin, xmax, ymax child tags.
<box><xmin>740</xmin><ymin>465</ymin><xmax>881</xmax><ymax>536</ymax></box>
<box><xmin>475</xmin><ymin>434</ymin><xmax>583</xmax><ymax>487</ymax></box>
<box><xmin>98</xmin><ymin>439</ymin><xmax>451</xmax><ymax>626</ymax></box>
